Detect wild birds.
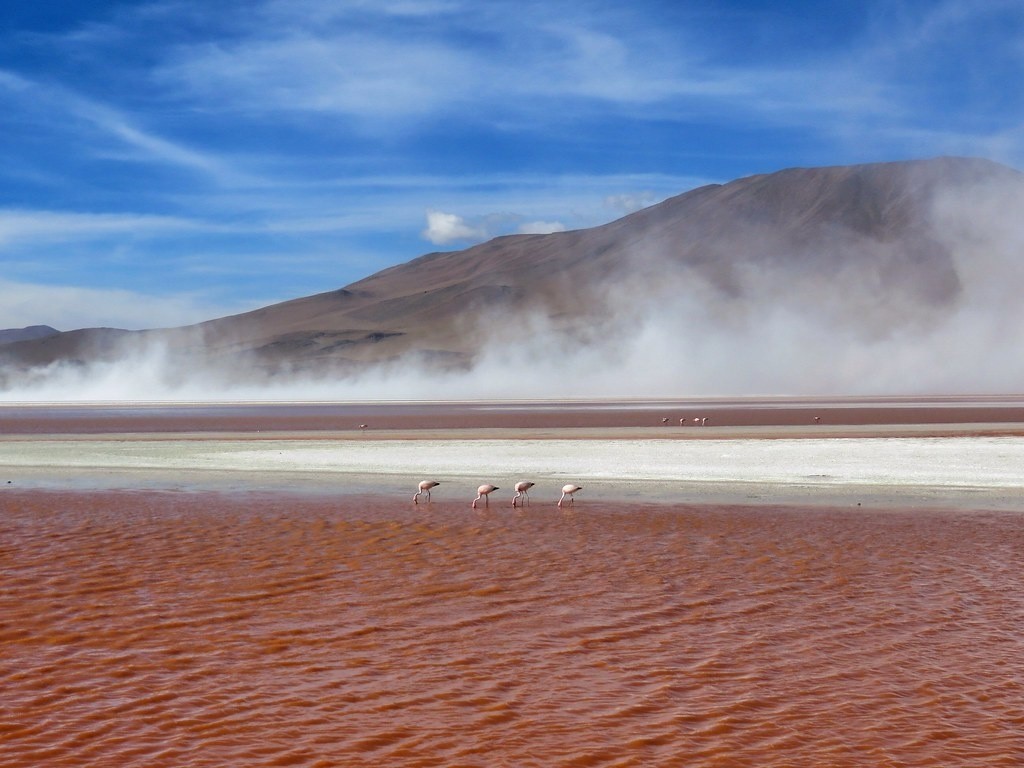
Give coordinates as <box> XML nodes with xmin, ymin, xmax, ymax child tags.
<box><xmin>813</xmin><ymin>416</ymin><xmax>820</xmax><ymax>423</ymax></box>
<box><xmin>359</xmin><ymin>424</ymin><xmax>368</xmax><ymax>430</ymax></box>
<box><xmin>512</xmin><ymin>481</ymin><xmax>535</xmax><ymax>508</ymax></box>
<box><xmin>473</xmin><ymin>484</ymin><xmax>500</xmax><ymax>508</ymax></box>
<box><xmin>412</xmin><ymin>480</ymin><xmax>441</xmax><ymax>505</ymax></box>
<box><xmin>662</xmin><ymin>416</ymin><xmax>707</xmax><ymax>426</ymax></box>
<box><xmin>558</xmin><ymin>485</ymin><xmax>583</xmax><ymax>508</ymax></box>
